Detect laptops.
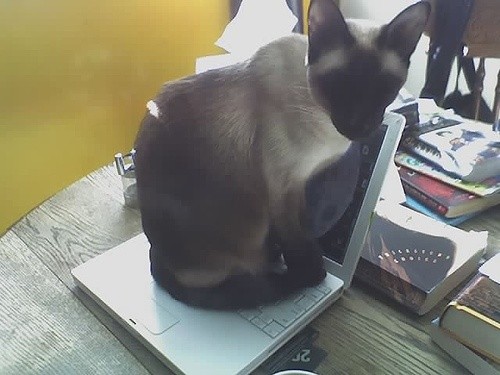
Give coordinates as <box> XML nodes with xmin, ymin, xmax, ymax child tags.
<box><xmin>69</xmin><ymin>111</ymin><xmax>408</xmax><ymax>371</ymax></box>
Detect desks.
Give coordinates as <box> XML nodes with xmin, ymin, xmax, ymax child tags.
<box><xmin>0</xmin><ymin>152</ymin><xmax>500</xmax><ymax>375</ymax></box>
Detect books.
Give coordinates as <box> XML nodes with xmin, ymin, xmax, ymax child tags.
<box><xmin>439</xmin><ymin>254</ymin><xmax>500</xmax><ymax>364</ymax></box>
<box><xmin>430</xmin><ymin>317</ymin><xmax>500</xmax><ymax>375</ymax></box>
<box><xmin>353</xmin><ymin>198</ymin><xmax>487</xmax><ymax>316</ymax></box>
<box><xmin>394</xmin><ymin>114</ymin><xmax>500</xmax><ymax>225</ymax></box>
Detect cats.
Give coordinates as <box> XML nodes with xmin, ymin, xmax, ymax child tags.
<box><xmin>133</xmin><ymin>0</ymin><xmax>431</xmax><ymax>311</ymax></box>
